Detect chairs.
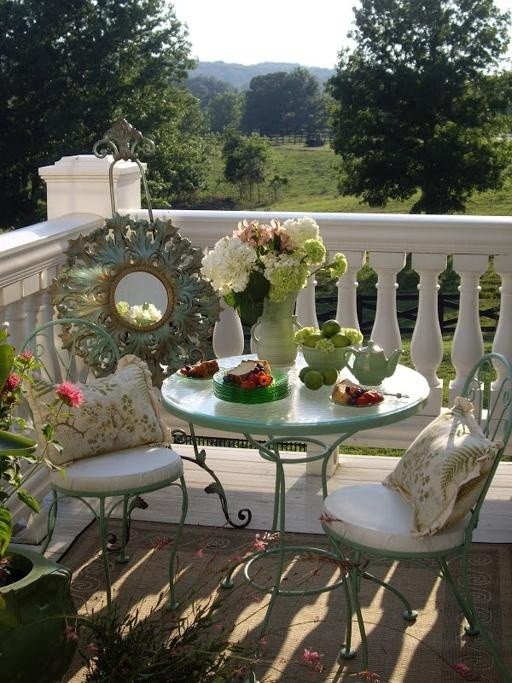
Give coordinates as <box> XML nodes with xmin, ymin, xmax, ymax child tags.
<box><xmin>17</xmin><ymin>320</ymin><xmax>188</xmax><ymax>617</ymax></box>
<box><xmin>320</xmin><ymin>352</ymin><xmax>511</xmax><ymax>683</ymax></box>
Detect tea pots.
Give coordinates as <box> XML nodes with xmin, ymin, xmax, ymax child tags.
<box><xmin>343</xmin><ymin>340</ymin><xmax>404</xmax><ymax>386</ymax></box>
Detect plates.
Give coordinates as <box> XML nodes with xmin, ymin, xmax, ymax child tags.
<box><xmin>329</xmin><ymin>395</ymin><xmax>385</xmax><ymax>411</ymax></box>
<box><xmin>176</xmin><ymin>366</ymin><xmax>229</xmax><ymax>380</ymax></box>
<box><xmin>211</xmin><ymin>368</ymin><xmax>291</xmax><ymax>404</ymax></box>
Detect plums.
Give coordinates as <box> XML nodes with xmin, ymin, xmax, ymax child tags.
<box><xmin>356</xmin><ymin>390</ymin><xmax>384</xmax><ymax>404</ymax></box>
<box><xmin>240</xmin><ymin>371</ymin><xmax>272</xmax><ymax>389</ymax></box>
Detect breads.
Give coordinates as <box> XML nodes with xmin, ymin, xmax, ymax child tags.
<box><xmin>227</xmin><ymin>360</ymin><xmax>270</xmax><ymax>384</ymax></box>
<box><xmin>332</xmin><ymin>379</ymin><xmax>362</xmax><ymax>404</ymax></box>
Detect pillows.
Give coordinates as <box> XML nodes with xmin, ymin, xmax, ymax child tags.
<box><xmin>20</xmin><ymin>352</ymin><xmax>171</xmax><ymax>469</ymax></box>
<box><xmin>382</xmin><ymin>395</ymin><xmax>503</xmax><ymax>538</ymax></box>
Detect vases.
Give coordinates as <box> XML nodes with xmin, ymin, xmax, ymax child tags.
<box><xmin>0</xmin><ymin>543</ymin><xmax>81</xmax><ymax>683</ymax></box>
<box><xmin>250</xmin><ymin>291</ymin><xmax>299</xmax><ymax>367</ymax></box>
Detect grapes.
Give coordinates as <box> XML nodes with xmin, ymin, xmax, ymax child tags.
<box><xmin>254</xmin><ymin>362</ymin><xmax>265</xmax><ymax>374</ymax></box>
<box><xmin>350</xmin><ymin>388</ymin><xmax>370</xmax><ymax>405</ymax></box>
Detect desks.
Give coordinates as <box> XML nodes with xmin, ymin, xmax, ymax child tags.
<box><xmin>160</xmin><ymin>353</ymin><xmax>431</xmax><ymax>660</ymax></box>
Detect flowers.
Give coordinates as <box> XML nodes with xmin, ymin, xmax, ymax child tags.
<box><xmin>203</xmin><ymin>217</ymin><xmax>348</xmax><ymax>301</ymax></box>
<box><xmin>0</xmin><ymin>321</ymin><xmax>83</xmax><ymax>584</ymax></box>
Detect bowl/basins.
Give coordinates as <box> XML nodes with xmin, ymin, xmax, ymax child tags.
<box><xmin>302</xmin><ymin>344</ymin><xmax>354</xmax><ymax>373</ymax></box>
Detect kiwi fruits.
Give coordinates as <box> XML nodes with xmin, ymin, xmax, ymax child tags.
<box><xmin>303</xmin><ymin>370</ymin><xmax>324</xmax><ymax>390</ymax></box>
<box><xmin>330</xmin><ymin>333</ymin><xmax>351</xmax><ymax>347</ymax></box>
<box><xmin>322</xmin><ymin>319</ymin><xmax>341</xmax><ymax>338</ymax></box>
<box><xmin>321</xmin><ymin>365</ymin><xmax>338</xmax><ymax>385</ymax></box>
<box><xmin>298</xmin><ymin>366</ymin><xmax>315</xmax><ymax>383</ymax></box>
